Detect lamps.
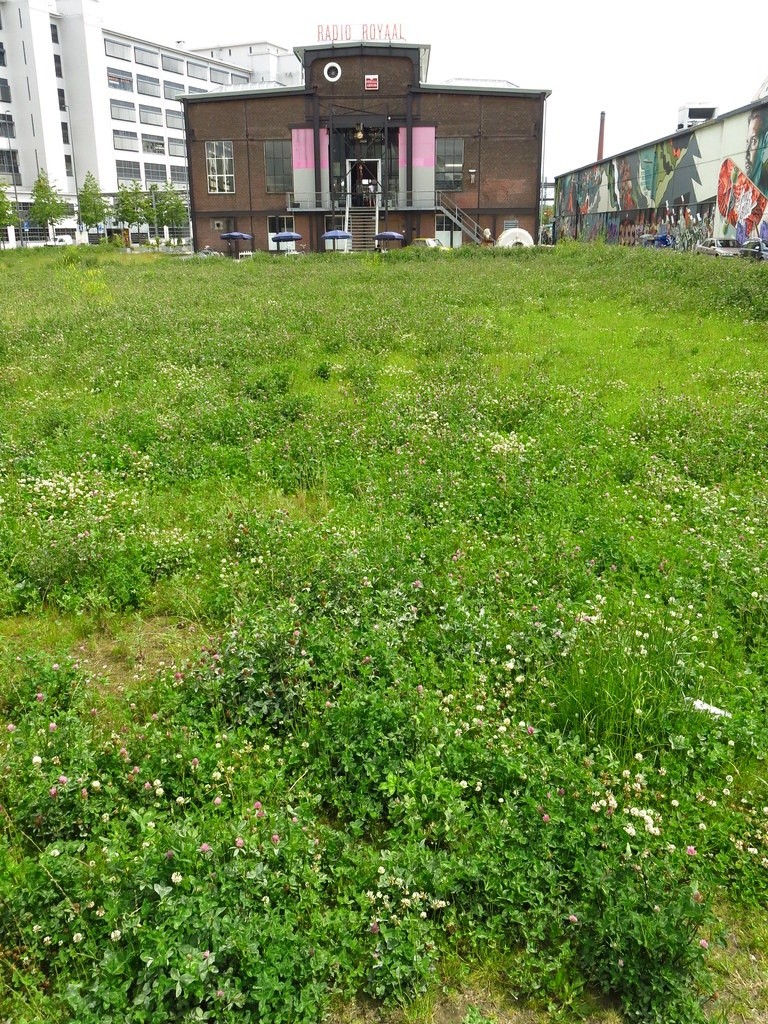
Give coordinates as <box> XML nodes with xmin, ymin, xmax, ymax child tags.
<box><xmin>353</xmin><ymin>122</ymin><xmax>365</xmax><ymax>141</ymax></box>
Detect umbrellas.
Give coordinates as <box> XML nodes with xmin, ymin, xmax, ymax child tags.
<box><xmin>220</xmin><ymin>231</ymin><xmax>253</xmax><ymax>258</ymax></box>
<box><xmin>321</xmin><ymin>229</ymin><xmax>353</xmax><ymax>253</ymax></box>
<box><xmin>272</xmin><ymin>232</ymin><xmax>302</xmax><ymax>256</ymax></box>
<box><xmin>372</xmin><ymin>231</ymin><xmax>405</xmax><ymax>253</ymax></box>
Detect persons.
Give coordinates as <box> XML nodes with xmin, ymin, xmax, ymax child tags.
<box><xmin>373</xmin><ymin>244</ymin><xmax>381</xmax><ymax>253</ymax></box>
<box><xmin>352</xmin><ymin>179</ymin><xmax>376</xmax><ymax>207</ymax></box>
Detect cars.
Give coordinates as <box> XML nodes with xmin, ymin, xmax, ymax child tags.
<box><xmin>740</xmin><ymin>240</ymin><xmax>768</xmax><ymax>262</ymax></box>
<box><xmin>696</xmin><ymin>237</ymin><xmax>743</xmax><ymax>261</ymax></box>
<box><xmin>44</xmin><ymin>237</ymin><xmax>68</xmax><ymax>245</ymax></box>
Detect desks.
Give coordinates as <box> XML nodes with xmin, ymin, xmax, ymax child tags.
<box><xmin>285</xmin><ymin>251</ymin><xmax>301</xmax><ymax>257</ymax></box>
<box><xmin>238</xmin><ymin>252</ymin><xmax>255</xmax><ymax>260</ymax></box>
